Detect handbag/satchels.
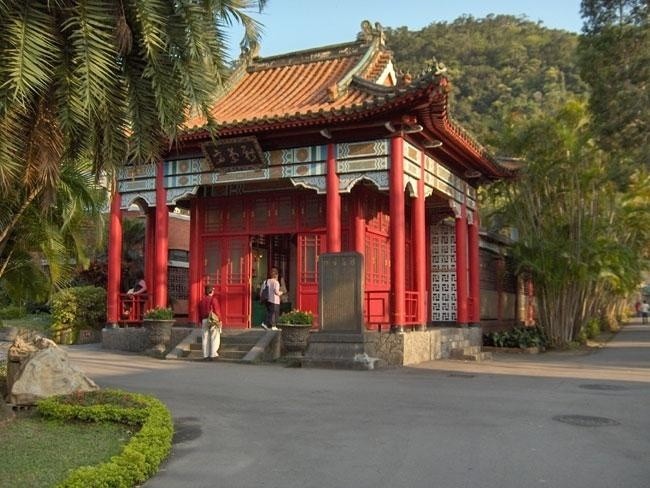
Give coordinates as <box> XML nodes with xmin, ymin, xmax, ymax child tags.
<box><xmin>207</xmin><ymin>297</ymin><xmax>221</xmax><ymax>328</ymax></box>
<box><xmin>260</xmin><ymin>279</ymin><xmax>269</xmax><ymax>304</ymax></box>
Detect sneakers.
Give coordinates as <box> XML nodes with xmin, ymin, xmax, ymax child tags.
<box><xmin>272</xmin><ymin>326</ymin><xmax>282</xmax><ymax>331</ymax></box>
<box><xmin>261</xmin><ymin>322</ymin><xmax>269</xmax><ymax>331</ymax></box>
<box><xmin>203</xmin><ymin>355</ymin><xmax>219</xmax><ymax>360</ymax></box>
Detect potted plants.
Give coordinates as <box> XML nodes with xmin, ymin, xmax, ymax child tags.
<box><xmin>276</xmin><ymin>308</ymin><xmax>314</xmax><ymax>351</ymax></box>
<box><xmin>144</xmin><ymin>305</ymin><xmax>176</xmax><ymax>345</ymax></box>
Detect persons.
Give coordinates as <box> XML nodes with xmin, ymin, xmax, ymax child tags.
<box><xmin>640</xmin><ymin>300</ymin><xmax>650</xmax><ymax>324</ymax></box>
<box><xmin>267</xmin><ymin>266</ymin><xmax>291</xmax><ymax>316</ymax></box>
<box><xmin>197</xmin><ymin>284</ymin><xmax>222</xmax><ymax>359</ymax></box>
<box><xmin>635</xmin><ymin>301</ymin><xmax>641</xmax><ymax>318</ymax></box>
<box><xmin>259</xmin><ymin>270</ymin><xmax>284</xmax><ymax>331</ymax></box>
<box><xmin>127</xmin><ymin>268</ymin><xmax>147</xmax><ymax>329</ymax></box>
